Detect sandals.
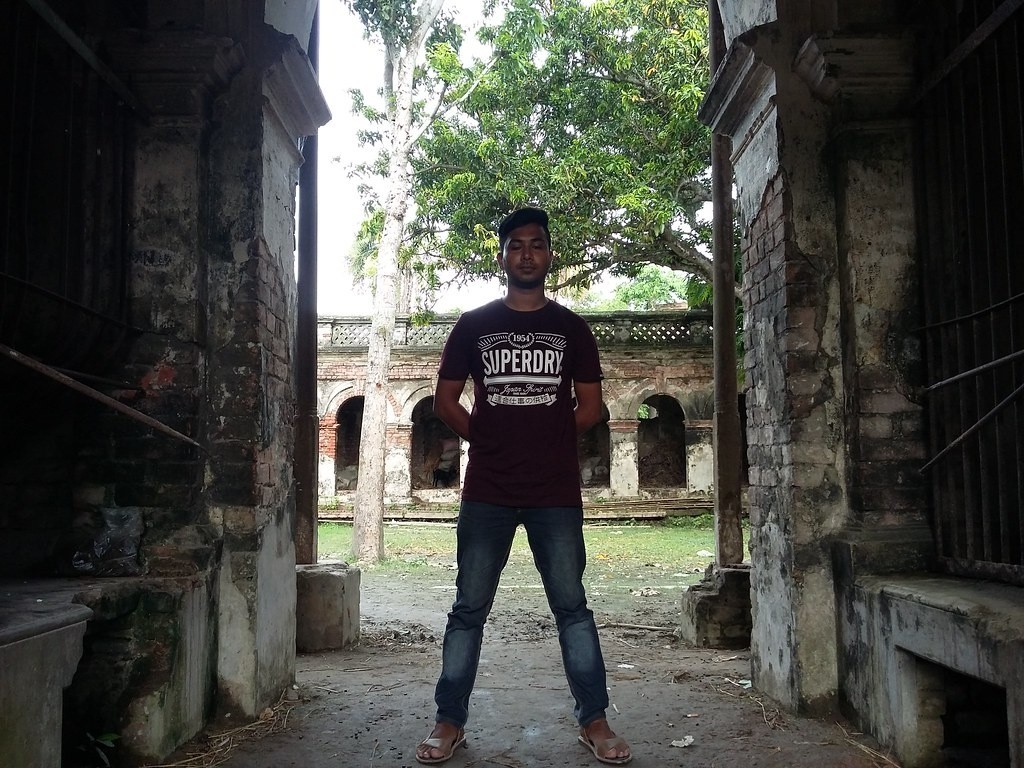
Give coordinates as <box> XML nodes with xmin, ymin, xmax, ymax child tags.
<box><xmin>416</xmin><ymin>718</ymin><xmax>467</xmax><ymax>764</ymax></box>
<box><xmin>577</xmin><ymin>717</ymin><xmax>632</xmax><ymax>764</ymax></box>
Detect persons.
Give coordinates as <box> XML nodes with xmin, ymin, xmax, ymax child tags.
<box><xmin>413</xmin><ymin>207</ymin><xmax>635</xmax><ymax>764</ymax></box>
